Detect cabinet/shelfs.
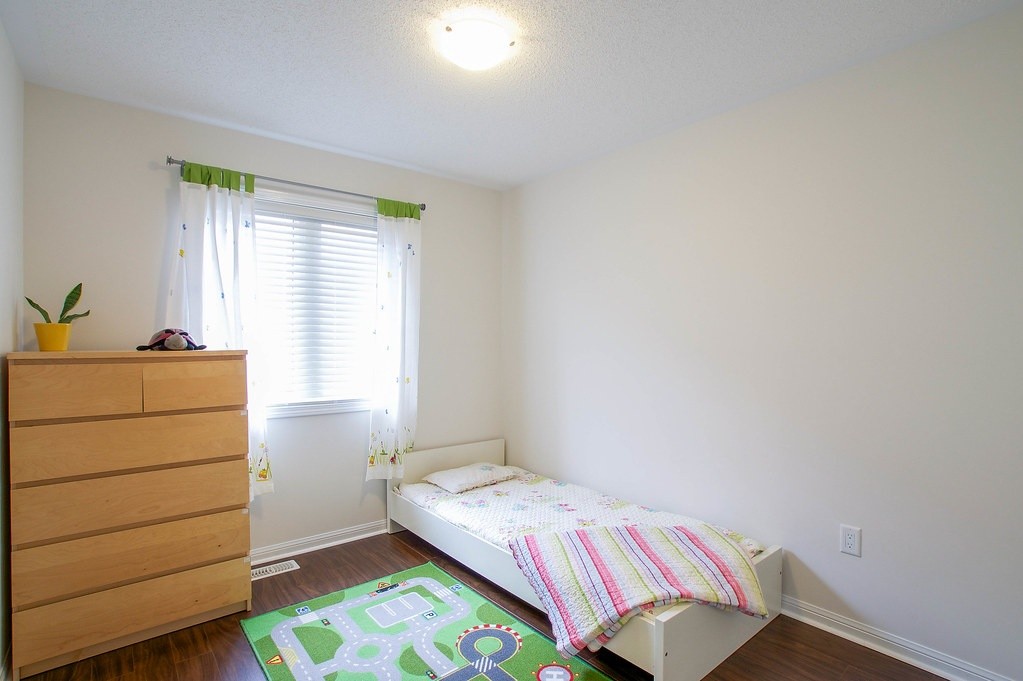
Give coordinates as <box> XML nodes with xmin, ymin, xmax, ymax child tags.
<box><xmin>6</xmin><ymin>350</ymin><xmax>251</xmax><ymax>681</ymax></box>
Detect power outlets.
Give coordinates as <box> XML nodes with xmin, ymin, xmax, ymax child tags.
<box><xmin>839</xmin><ymin>524</ymin><xmax>863</xmax><ymax>558</ymax></box>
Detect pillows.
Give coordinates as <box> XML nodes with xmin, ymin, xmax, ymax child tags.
<box><xmin>422</xmin><ymin>462</ymin><xmax>515</xmax><ymax>495</ymax></box>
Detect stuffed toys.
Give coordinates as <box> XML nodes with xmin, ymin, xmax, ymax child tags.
<box><xmin>135</xmin><ymin>327</ymin><xmax>207</xmax><ymax>352</ymax></box>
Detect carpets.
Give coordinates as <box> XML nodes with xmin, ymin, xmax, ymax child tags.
<box><xmin>239</xmin><ymin>560</ymin><xmax>619</xmax><ymax>680</ymax></box>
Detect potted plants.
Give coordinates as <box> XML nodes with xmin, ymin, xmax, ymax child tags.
<box><xmin>24</xmin><ymin>283</ymin><xmax>91</xmax><ymax>352</ymax></box>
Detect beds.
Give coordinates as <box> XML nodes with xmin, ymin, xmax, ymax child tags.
<box><xmin>386</xmin><ymin>438</ymin><xmax>782</xmax><ymax>681</ymax></box>
